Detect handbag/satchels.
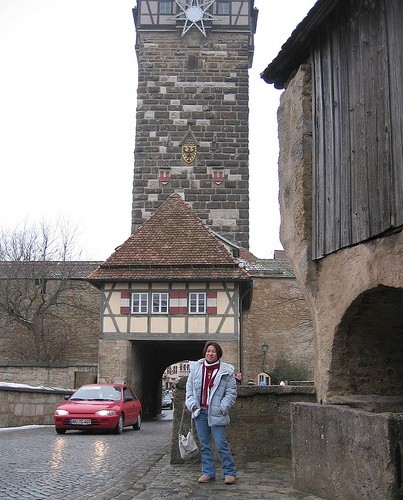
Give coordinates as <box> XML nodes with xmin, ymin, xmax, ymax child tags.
<box><xmin>178</xmin><ymin>429</ymin><xmax>199</xmax><ymax>460</ymax></box>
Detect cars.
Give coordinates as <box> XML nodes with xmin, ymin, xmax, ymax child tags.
<box><xmin>162</xmin><ymin>396</ymin><xmax>173</xmax><ymax>410</ymax></box>
<box><xmin>54</xmin><ymin>383</ymin><xmax>141</xmax><ymax>435</ymax></box>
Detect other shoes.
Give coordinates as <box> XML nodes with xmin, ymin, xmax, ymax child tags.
<box><xmin>198</xmin><ymin>475</ymin><xmax>214</xmax><ymax>482</ymax></box>
<box><xmin>223</xmin><ymin>474</ymin><xmax>236</xmax><ymax>484</ymax></box>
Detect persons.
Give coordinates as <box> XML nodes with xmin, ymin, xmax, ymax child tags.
<box><xmin>186</xmin><ymin>341</ymin><xmax>238</xmax><ymax>484</ymax></box>
<box><xmin>280</xmin><ymin>379</ymin><xmax>285</xmax><ymax>386</ymax></box>
<box><xmin>258</xmin><ymin>381</ymin><xmax>267</xmax><ymax>386</ymax></box>
<box><xmin>233</xmin><ymin>372</ymin><xmax>242</xmax><ymax>385</ymax></box>
<box><xmin>247</xmin><ymin>379</ymin><xmax>255</xmax><ymax>385</ymax></box>
